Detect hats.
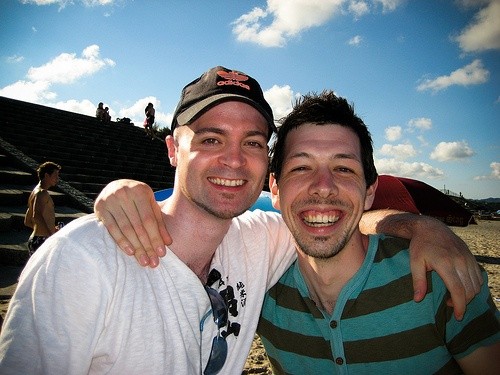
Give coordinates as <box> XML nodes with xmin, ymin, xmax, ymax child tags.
<box><xmin>170</xmin><ymin>66</ymin><xmax>274</xmax><ymax>143</ymax></box>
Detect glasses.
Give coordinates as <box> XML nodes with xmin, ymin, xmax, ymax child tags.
<box><xmin>201</xmin><ymin>285</ymin><xmax>228</xmax><ymax>375</ymax></box>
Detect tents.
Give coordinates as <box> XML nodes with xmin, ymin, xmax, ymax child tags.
<box><xmin>152</xmin><ymin>188</ymin><xmax>282</xmax><ymax>214</ymax></box>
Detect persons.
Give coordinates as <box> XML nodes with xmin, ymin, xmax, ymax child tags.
<box><xmin>0</xmin><ymin>66</ymin><xmax>484</xmax><ymax>375</ymax></box>
<box><xmin>94</xmin><ymin>89</ymin><xmax>500</xmax><ymax>375</ymax></box>
<box><xmin>103</xmin><ymin>107</ymin><xmax>111</xmax><ymax>122</ymax></box>
<box><xmin>143</xmin><ymin>102</ymin><xmax>155</xmax><ymax>129</ymax></box>
<box><xmin>96</xmin><ymin>102</ymin><xmax>105</xmax><ymax>121</ymax></box>
<box><xmin>23</xmin><ymin>161</ymin><xmax>61</xmax><ymax>258</ymax></box>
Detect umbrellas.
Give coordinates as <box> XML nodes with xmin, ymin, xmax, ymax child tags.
<box><xmin>363</xmin><ymin>175</ymin><xmax>479</xmax><ymax>228</ymax></box>
